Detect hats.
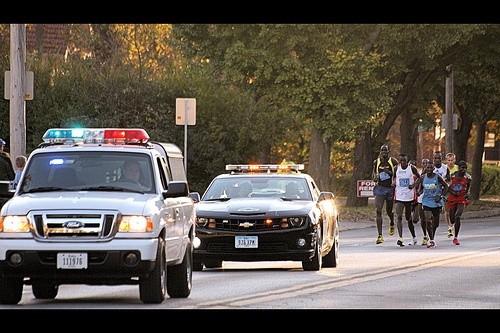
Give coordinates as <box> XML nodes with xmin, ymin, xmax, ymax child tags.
<box><xmin>0</xmin><ymin>139</ymin><xmax>6</xmax><ymax>145</ymax></box>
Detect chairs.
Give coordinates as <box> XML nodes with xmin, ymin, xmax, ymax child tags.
<box><xmin>51</xmin><ymin>167</ymin><xmax>85</xmax><ymax>186</ymax></box>
<box><xmin>230</xmin><ymin>187</ymin><xmax>240</xmax><ymax>197</ymax></box>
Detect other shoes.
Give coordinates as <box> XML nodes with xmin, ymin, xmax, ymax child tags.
<box><xmin>448</xmin><ymin>229</ymin><xmax>452</xmax><ymax>236</ymax></box>
<box><xmin>453</xmin><ymin>239</ymin><xmax>459</xmax><ymax>244</ymax></box>
<box><xmin>422</xmin><ymin>236</ymin><xmax>429</xmax><ymax>245</ymax></box>
<box><xmin>413</xmin><ymin>237</ymin><xmax>417</xmax><ymax>244</ymax></box>
<box><xmin>397</xmin><ymin>238</ymin><xmax>404</xmax><ymax>246</ymax></box>
<box><xmin>427</xmin><ymin>240</ymin><xmax>435</xmax><ymax>247</ymax></box>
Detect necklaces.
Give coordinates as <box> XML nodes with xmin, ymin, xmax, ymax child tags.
<box><xmin>237</xmin><ymin>182</ymin><xmax>252</xmax><ymax>198</ymax></box>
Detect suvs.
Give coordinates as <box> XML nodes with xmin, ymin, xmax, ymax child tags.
<box><xmin>0</xmin><ymin>125</ymin><xmax>196</xmax><ymax>305</ymax></box>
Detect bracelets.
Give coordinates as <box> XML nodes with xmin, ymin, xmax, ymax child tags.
<box><xmin>389</xmin><ymin>170</ymin><xmax>391</xmax><ymax>175</ymax></box>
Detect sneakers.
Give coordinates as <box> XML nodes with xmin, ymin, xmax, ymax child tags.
<box><xmin>389</xmin><ymin>225</ymin><xmax>395</xmax><ymax>235</ymax></box>
<box><xmin>376</xmin><ymin>236</ymin><xmax>384</xmax><ymax>243</ymax></box>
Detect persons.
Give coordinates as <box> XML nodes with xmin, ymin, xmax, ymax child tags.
<box><xmin>119</xmin><ymin>158</ymin><xmax>150</xmax><ymax>189</ymax></box>
<box><xmin>0</xmin><ymin>138</ymin><xmax>31</xmax><ymax>197</ymax></box>
<box><xmin>447</xmin><ymin>161</ymin><xmax>472</xmax><ymax>245</ymax></box>
<box><xmin>285</xmin><ymin>182</ymin><xmax>300</xmax><ymax>196</ymax></box>
<box><xmin>419</xmin><ymin>162</ymin><xmax>448</xmax><ymax>247</ymax></box>
<box><xmin>409</xmin><ymin>151</ymin><xmax>472</xmax><ymax>246</ymax></box>
<box><xmin>390</xmin><ymin>153</ymin><xmax>421</xmax><ymax>246</ymax></box>
<box><xmin>373</xmin><ymin>144</ymin><xmax>399</xmax><ymax>244</ymax></box>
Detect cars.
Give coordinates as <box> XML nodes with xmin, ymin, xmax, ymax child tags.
<box><xmin>187</xmin><ymin>164</ymin><xmax>340</xmax><ymax>272</ymax></box>
